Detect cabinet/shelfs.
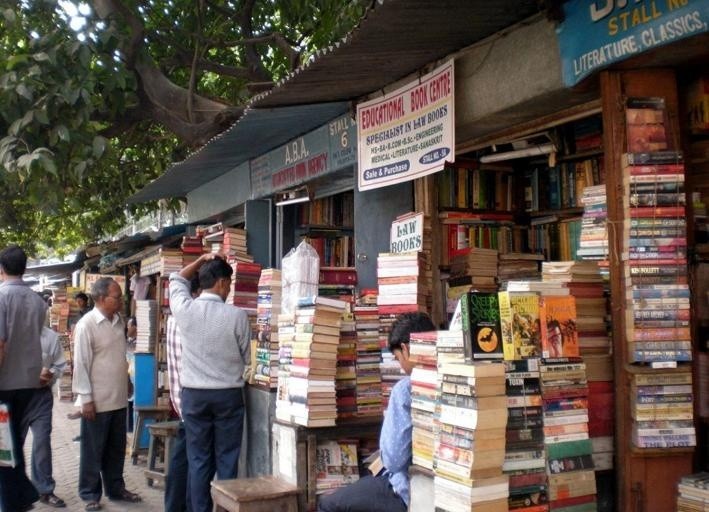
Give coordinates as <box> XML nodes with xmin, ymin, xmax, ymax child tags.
<box><xmin>413</xmin><ymin>136</ymin><xmax>604</xmax><ymax>336</ymax></box>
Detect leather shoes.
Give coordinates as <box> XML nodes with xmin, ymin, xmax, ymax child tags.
<box><xmin>38</xmin><ymin>491</ymin><xmax>67</xmax><ymax>508</ymax></box>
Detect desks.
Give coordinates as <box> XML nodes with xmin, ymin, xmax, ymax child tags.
<box><xmin>267</xmin><ymin>409</ymin><xmax>385</xmax><ymax>512</ymax></box>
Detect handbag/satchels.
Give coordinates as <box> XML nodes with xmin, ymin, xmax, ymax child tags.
<box><xmin>0</xmin><ymin>403</ymin><xmax>17</xmax><ymax>469</ymax></box>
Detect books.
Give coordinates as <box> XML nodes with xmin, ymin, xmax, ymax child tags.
<box><xmin>135</xmin><ymin>96</ymin><xmax>709</xmax><ymax>512</ymax></box>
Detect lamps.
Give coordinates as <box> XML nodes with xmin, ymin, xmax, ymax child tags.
<box><xmin>274</xmin><ymin>185</ymin><xmax>315</xmax><ymax>206</ymax></box>
<box><xmin>479</xmin><ymin>125</ymin><xmax>557</xmax><ymax>164</ymax></box>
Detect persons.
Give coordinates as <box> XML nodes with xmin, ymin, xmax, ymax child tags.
<box><xmin>0</xmin><ymin>246</ymin><xmax>141</xmax><ymax>512</ymax></box>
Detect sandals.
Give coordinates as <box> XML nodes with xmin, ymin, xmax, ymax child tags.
<box><xmin>82</xmin><ymin>498</ymin><xmax>101</xmax><ymax>512</ymax></box>
<box><xmin>109</xmin><ymin>487</ymin><xmax>141</xmax><ymax>503</ymax></box>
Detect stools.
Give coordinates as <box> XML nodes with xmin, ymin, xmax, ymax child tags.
<box><xmin>145</xmin><ymin>421</ymin><xmax>182</xmax><ymax>486</ymax></box>
<box><xmin>210</xmin><ymin>474</ymin><xmax>304</xmax><ymax>512</ymax></box>
<box><xmin>131</xmin><ymin>405</ymin><xmax>173</xmax><ymax>465</ymax></box>
